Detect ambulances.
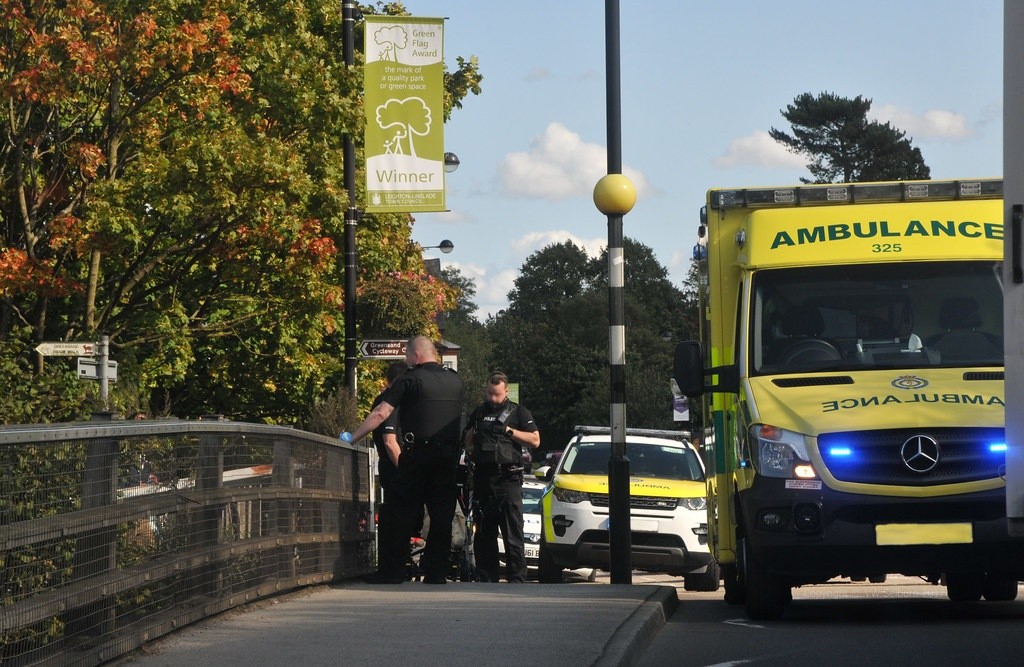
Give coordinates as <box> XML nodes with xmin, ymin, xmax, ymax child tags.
<box><xmin>535</xmin><ymin>424</ymin><xmax>720</xmax><ymax>592</ymax></box>
<box><xmin>692</xmin><ymin>176</ymin><xmax>1024</xmax><ymax>623</ymax></box>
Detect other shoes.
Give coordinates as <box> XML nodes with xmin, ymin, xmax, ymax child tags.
<box><xmin>363</xmin><ymin>571</ymin><xmax>404</xmax><ymax>584</ymax></box>
<box><xmin>509</xmin><ymin>576</ymin><xmax>523</xmax><ymax>584</ymax></box>
<box><xmin>474</xmin><ymin>571</ymin><xmax>499</xmax><ymax>582</ymax></box>
<box><xmin>423</xmin><ymin>575</ymin><xmax>447</xmax><ymax>585</ymax></box>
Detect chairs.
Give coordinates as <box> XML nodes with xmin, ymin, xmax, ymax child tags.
<box><xmin>929</xmin><ymin>295</ymin><xmax>994</xmax><ymax>358</ymax></box>
<box><xmin>773</xmin><ymin>305</ymin><xmax>838</xmax><ymax>357</ymax></box>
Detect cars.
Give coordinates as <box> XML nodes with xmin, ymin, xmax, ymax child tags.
<box><xmin>468</xmin><ymin>474</ymin><xmax>550</xmax><ymax>580</ymax></box>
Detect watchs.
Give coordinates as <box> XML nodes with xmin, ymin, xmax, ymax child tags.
<box><xmin>506</xmin><ymin>427</ymin><xmax>514</xmax><ymax>437</ymax></box>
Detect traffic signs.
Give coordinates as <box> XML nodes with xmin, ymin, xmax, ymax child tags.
<box><xmin>356</xmin><ymin>340</ymin><xmax>408</xmax><ymax>362</ymax></box>
<box><xmin>34</xmin><ymin>340</ymin><xmax>99</xmax><ymax>357</ymax></box>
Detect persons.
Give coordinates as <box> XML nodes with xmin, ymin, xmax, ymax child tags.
<box><xmin>339</xmin><ymin>335</ymin><xmax>463</xmax><ymax>584</ymax></box>
<box><xmin>465</xmin><ymin>371</ymin><xmax>540</xmax><ymax>585</ymax></box>
